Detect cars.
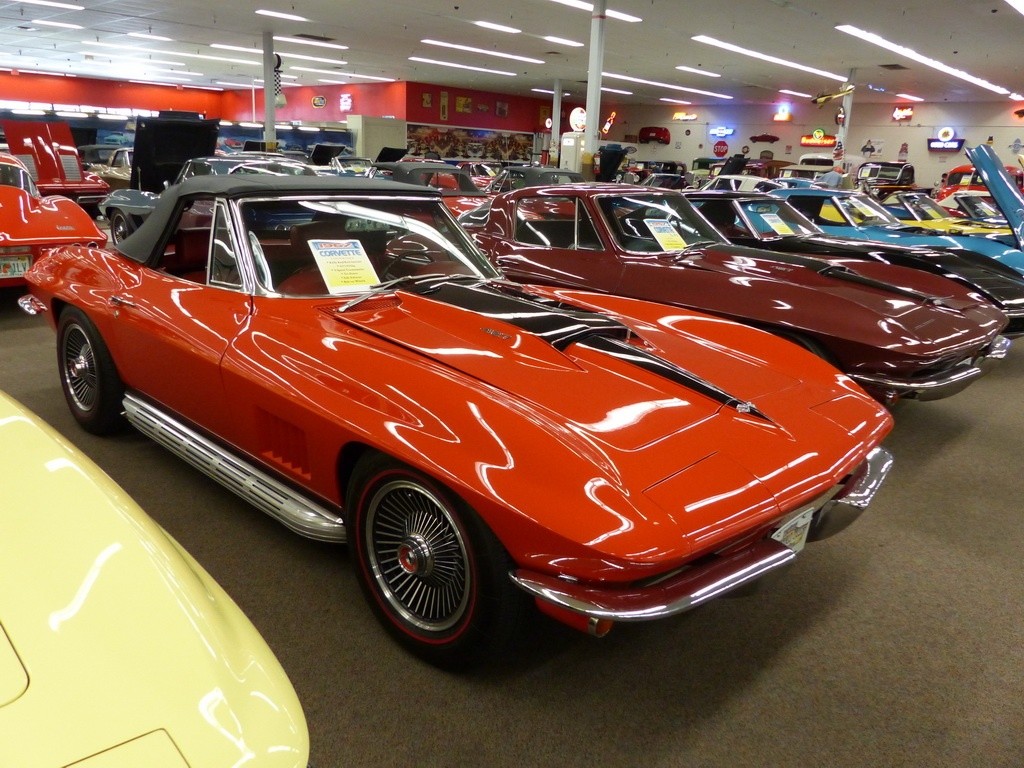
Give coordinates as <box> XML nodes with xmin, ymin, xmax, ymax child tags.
<box><xmin>0</xmin><ymin>117</ymin><xmax>1024</xmax><ymax>412</ymax></box>
<box><xmin>15</xmin><ymin>171</ymin><xmax>899</xmax><ymax>675</ymax></box>
